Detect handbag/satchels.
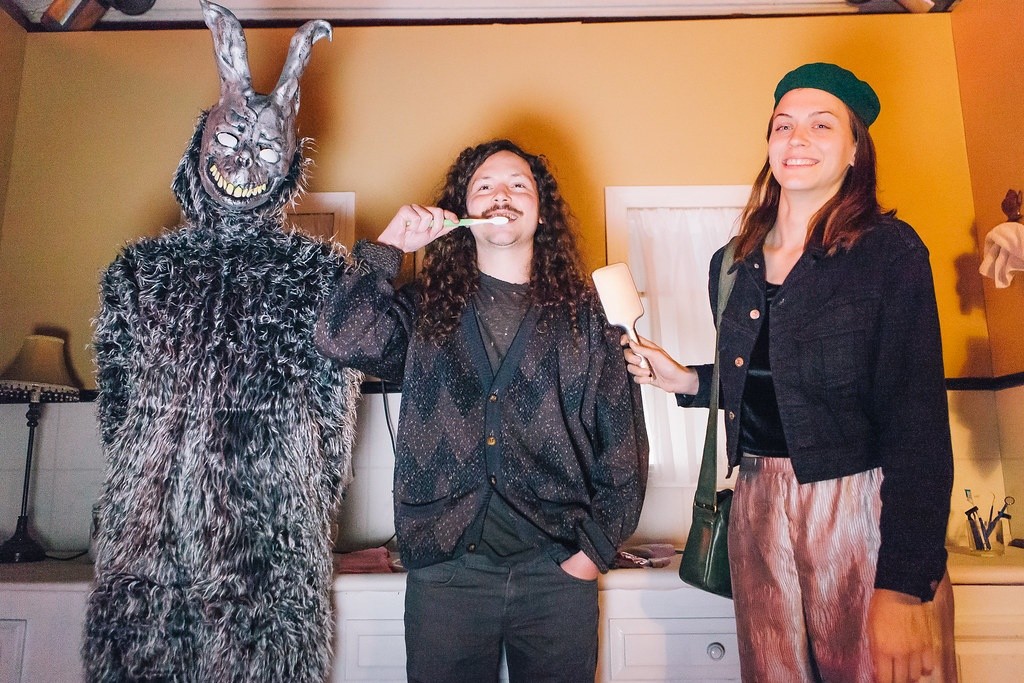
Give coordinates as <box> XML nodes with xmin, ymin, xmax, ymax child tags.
<box><xmin>678</xmin><ymin>489</ymin><xmax>733</xmax><ymax>602</ymax></box>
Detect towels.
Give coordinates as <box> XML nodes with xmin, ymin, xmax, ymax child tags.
<box><xmin>338</xmin><ymin>545</ymin><xmax>394</xmax><ymax>575</ymax></box>
<box><xmin>978</xmin><ymin>222</ymin><xmax>1024</xmax><ymax>288</ymax></box>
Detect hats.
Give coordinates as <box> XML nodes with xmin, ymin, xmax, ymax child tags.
<box><xmin>773</xmin><ymin>62</ymin><xmax>881</xmax><ymax>126</ymax></box>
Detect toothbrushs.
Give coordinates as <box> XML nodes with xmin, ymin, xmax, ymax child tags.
<box><xmin>406</xmin><ymin>215</ymin><xmax>510</xmax><ymax>226</ymax></box>
<box><xmin>965</xmin><ymin>488</ymin><xmax>991</xmax><ymax>550</ymax></box>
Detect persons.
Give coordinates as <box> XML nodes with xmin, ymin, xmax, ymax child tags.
<box><xmin>618</xmin><ymin>63</ymin><xmax>959</xmax><ymax>683</ymax></box>
<box><xmin>83</xmin><ymin>0</ymin><xmax>366</xmax><ymax>683</ymax></box>
<box><xmin>313</xmin><ymin>139</ymin><xmax>649</xmax><ymax>683</ymax></box>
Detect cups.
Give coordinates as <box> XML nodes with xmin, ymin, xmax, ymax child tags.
<box><xmin>966</xmin><ymin>520</ymin><xmax>1005</xmax><ymax>558</ymax></box>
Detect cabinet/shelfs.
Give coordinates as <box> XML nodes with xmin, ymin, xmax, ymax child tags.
<box><xmin>0</xmin><ymin>586</ymin><xmax>1024</xmax><ymax>683</ymax></box>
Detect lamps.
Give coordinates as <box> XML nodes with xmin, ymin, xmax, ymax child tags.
<box><xmin>0</xmin><ymin>335</ymin><xmax>83</xmax><ymax>562</ymax></box>
